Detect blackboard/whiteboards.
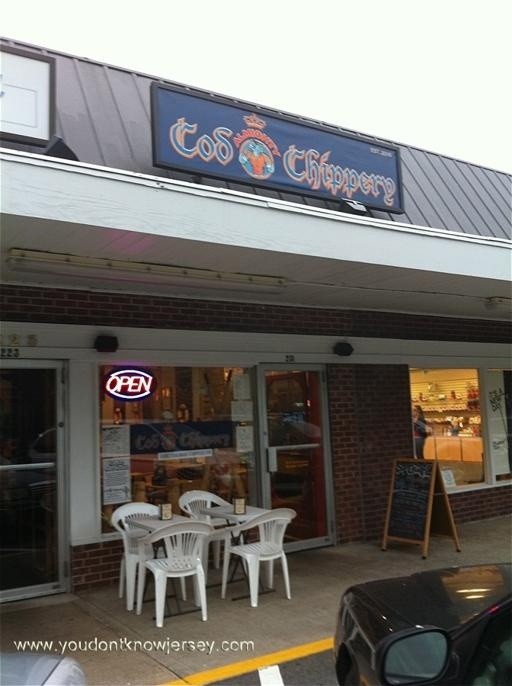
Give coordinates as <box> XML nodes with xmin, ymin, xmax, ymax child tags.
<box><xmin>384</xmin><ymin>459</ymin><xmax>437</xmax><ymax>545</ymax></box>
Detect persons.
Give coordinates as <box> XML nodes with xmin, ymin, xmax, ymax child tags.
<box><xmin>412</xmin><ymin>405</ymin><xmax>427</xmax><ymax>458</ymax></box>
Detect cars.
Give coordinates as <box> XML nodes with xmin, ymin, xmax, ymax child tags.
<box><xmin>333</xmin><ymin>562</ymin><xmax>512</xmax><ymax>685</ymax></box>
<box><xmin>24</xmin><ymin>415</ymin><xmax>323</xmax><ymax>506</ymax></box>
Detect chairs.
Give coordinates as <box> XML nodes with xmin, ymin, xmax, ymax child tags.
<box><xmin>110</xmin><ymin>489</ymin><xmax>300</xmax><ymax>628</ymax></box>
